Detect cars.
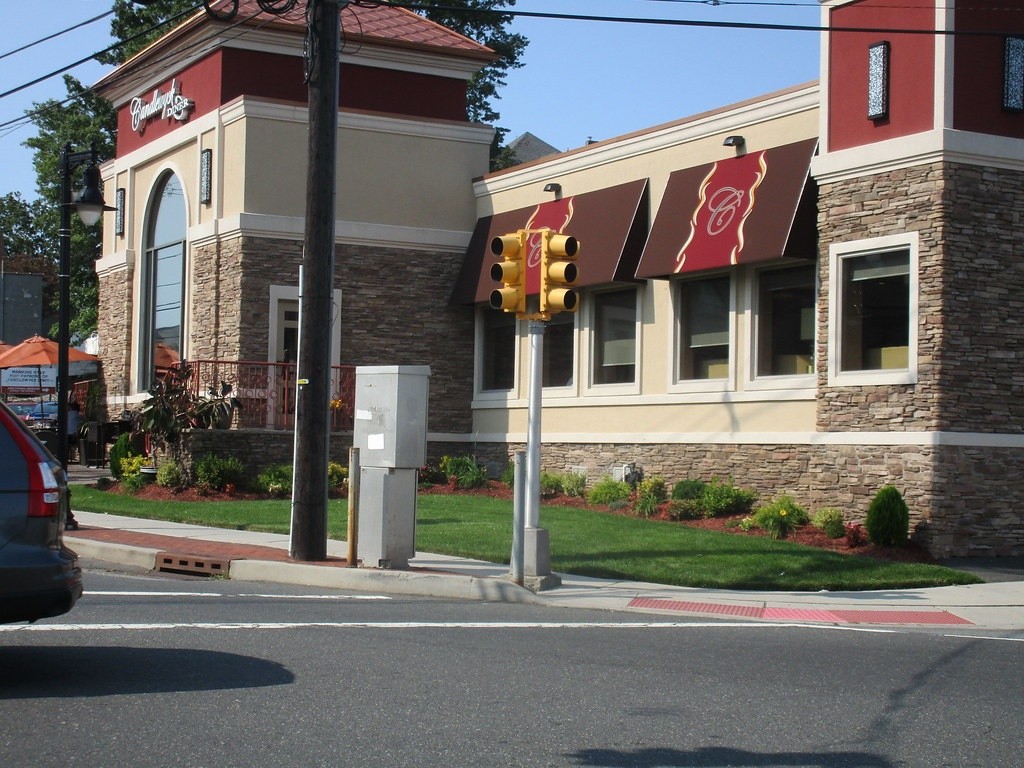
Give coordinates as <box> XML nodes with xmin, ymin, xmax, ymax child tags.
<box><xmin>6</xmin><ymin>401</ymin><xmax>38</xmax><ymax>423</ymax></box>
<box><xmin>26</xmin><ymin>400</ymin><xmax>59</xmax><ymax>427</ymax></box>
<box><xmin>0</xmin><ymin>392</ymin><xmax>84</xmax><ymax>626</ymax></box>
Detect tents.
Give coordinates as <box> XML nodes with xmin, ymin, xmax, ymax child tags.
<box><xmin>153</xmin><ymin>342</ymin><xmax>179</xmax><ymax>370</ymax></box>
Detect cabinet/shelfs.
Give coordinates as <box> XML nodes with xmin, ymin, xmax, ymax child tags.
<box><xmin>87</xmin><ymin>422</ymin><xmax>103</xmax><ymax>469</ymax></box>
<box><xmin>103</xmin><ymin>421</ymin><xmax>132</xmax><ymax>469</ymax></box>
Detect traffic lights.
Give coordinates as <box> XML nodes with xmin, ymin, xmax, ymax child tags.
<box><xmin>489</xmin><ymin>231</ymin><xmax>526</xmax><ymax>314</ymax></box>
<box><xmin>542</xmin><ymin>229</ymin><xmax>582</xmax><ymax>314</ymax></box>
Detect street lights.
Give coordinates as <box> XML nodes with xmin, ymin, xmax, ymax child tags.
<box><xmin>55</xmin><ymin>140</ymin><xmax>120</xmax><ymax>531</ymax></box>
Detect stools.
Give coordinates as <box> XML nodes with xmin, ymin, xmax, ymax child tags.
<box><xmin>68</xmin><ymin>444</ymin><xmax>80</xmax><ymax>462</ymax></box>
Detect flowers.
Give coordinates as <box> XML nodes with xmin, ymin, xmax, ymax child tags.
<box><xmin>330</xmin><ymin>400</ymin><xmax>348</xmax><ymax>412</ymax></box>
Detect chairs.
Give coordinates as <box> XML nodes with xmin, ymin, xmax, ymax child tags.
<box><xmin>33</xmin><ymin>431</ymin><xmax>59</xmax><ymax>451</ymax></box>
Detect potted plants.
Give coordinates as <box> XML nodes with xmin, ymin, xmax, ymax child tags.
<box><xmin>189</xmin><ymin>396</ymin><xmax>211</xmax><ymax>429</ymax></box>
<box><xmin>216</xmin><ymin>459</ymin><xmax>243</xmax><ymax>497</ymax></box>
<box><xmin>208</xmin><ymin>381</ymin><xmax>243</xmax><ymax>430</ymax></box>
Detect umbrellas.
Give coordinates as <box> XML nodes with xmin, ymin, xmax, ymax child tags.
<box><xmin>0</xmin><ymin>335</ymin><xmax>100</xmax><ymax>431</ymax></box>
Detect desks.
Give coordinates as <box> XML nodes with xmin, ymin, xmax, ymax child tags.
<box><xmin>31</xmin><ymin>427</ymin><xmax>58</xmax><ymax>432</ymax></box>
